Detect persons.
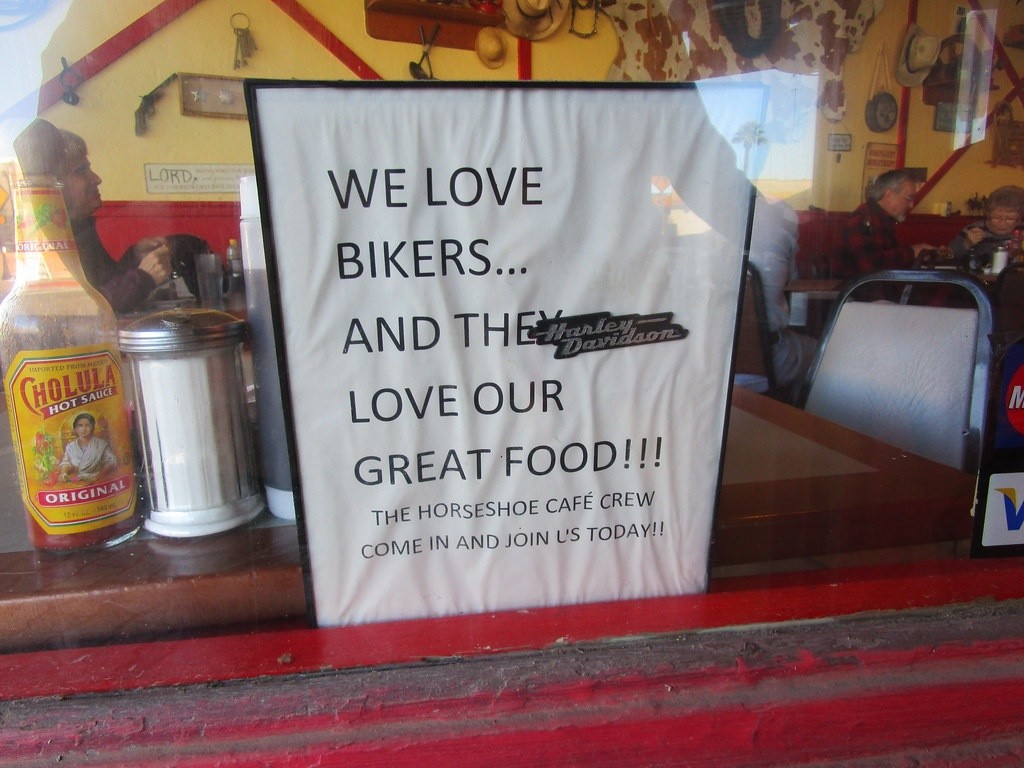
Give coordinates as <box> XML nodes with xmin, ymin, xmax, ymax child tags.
<box><xmin>952</xmin><ymin>185</ymin><xmax>1024</xmax><ymax>262</ymax></box>
<box><xmin>832</xmin><ymin>170</ymin><xmax>945</xmax><ymax>306</ymax></box>
<box><xmin>0</xmin><ymin>118</ymin><xmax>172</xmax><ymax>314</ymax></box>
<box><xmin>734</xmin><ymin>194</ymin><xmax>817</xmax><ymax>403</ymax></box>
<box><xmin>119</xmin><ymin>235</ymin><xmax>229</xmax><ymax>296</ymax></box>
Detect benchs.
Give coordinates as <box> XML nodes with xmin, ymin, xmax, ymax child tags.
<box><xmin>794</xmin><ymin>210</ymin><xmax>986</xmax><ymax>280</ymax></box>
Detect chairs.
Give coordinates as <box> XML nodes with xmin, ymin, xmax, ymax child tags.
<box><xmin>796</xmin><ymin>269</ymin><xmax>997</xmax><ymax>559</ymax></box>
<box><xmin>993</xmin><ymin>263</ymin><xmax>1024</xmax><ymax>335</ymax></box>
<box><xmin>733</xmin><ymin>261</ymin><xmax>778</xmax><ymax>400</ymax></box>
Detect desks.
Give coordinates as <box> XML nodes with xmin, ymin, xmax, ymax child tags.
<box><xmin>784</xmin><ymin>278</ymin><xmax>845</xmax><ymax>326</ymax></box>
<box><xmin>0</xmin><ymin>291</ymin><xmax>979</xmax><ymax>651</ymax></box>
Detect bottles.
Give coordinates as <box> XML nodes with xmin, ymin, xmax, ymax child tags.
<box><xmin>0</xmin><ymin>155</ymin><xmax>144</xmax><ymax>556</ymax></box>
<box><xmin>991</xmin><ymin>246</ymin><xmax>1008</xmax><ymax>275</ymax></box>
<box><xmin>226</xmin><ymin>239</ymin><xmax>244</xmax><ymax>293</ymax></box>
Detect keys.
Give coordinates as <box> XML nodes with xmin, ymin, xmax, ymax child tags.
<box><xmin>233</xmin><ymin>28</ymin><xmax>257</xmax><ymax>69</ymax></box>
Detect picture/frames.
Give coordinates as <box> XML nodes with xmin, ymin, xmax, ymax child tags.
<box><xmin>179</xmin><ymin>72</ymin><xmax>248</xmax><ymax>119</ymax></box>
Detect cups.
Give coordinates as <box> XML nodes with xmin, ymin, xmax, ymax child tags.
<box><xmin>193</xmin><ymin>252</ymin><xmax>225</xmax><ymax>310</ymax></box>
<box><xmin>239</xmin><ymin>175</ymin><xmax>296</xmax><ymax>521</ymax></box>
<box><xmin>120</xmin><ymin>304</ymin><xmax>265</xmax><ymax>539</ymax></box>
